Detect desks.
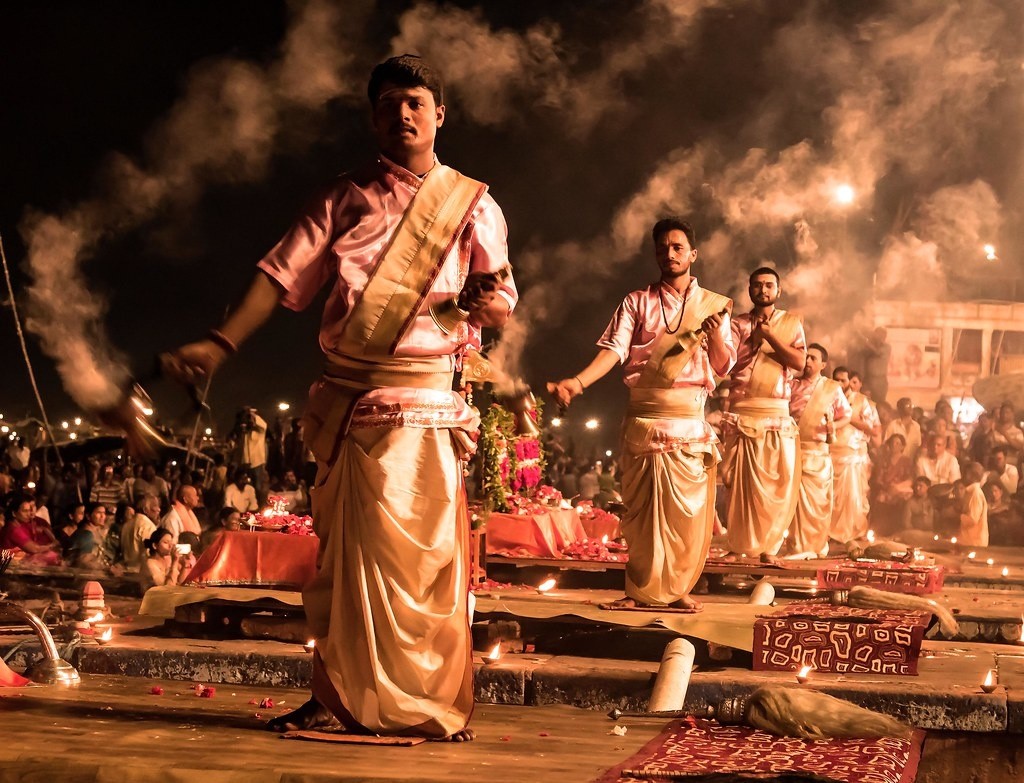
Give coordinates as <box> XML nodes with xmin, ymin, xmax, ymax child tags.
<box><xmin>486</xmin><ymin>508</ymin><xmax>580</xmax><ymax>553</ymax></box>
<box><xmin>192</xmin><ymin>529</ymin><xmax>319</xmax><ymax>587</ymax></box>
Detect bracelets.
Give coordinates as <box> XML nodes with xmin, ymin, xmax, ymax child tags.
<box><xmin>574</xmin><ymin>374</ymin><xmax>587</xmax><ymax>397</ymax></box>
<box><xmin>209</xmin><ymin>327</ymin><xmax>238</xmax><ymax>361</ymax></box>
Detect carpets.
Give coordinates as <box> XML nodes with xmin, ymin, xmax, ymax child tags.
<box><xmin>591</xmin><ymin>546</ymin><xmax>944</xmax><ymax>783</ymax></box>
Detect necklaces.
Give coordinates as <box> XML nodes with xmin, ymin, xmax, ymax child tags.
<box><xmin>652</xmin><ymin>284</ymin><xmax>690</xmax><ymax>334</ymax></box>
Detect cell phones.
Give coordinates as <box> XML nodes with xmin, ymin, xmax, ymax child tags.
<box><xmin>176</xmin><ymin>544</ymin><xmax>191</xmax><ymax>555</ymax></box>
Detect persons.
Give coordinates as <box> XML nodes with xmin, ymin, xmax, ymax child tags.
<box><xmin>1</xmin><ymin>379</ymin><xmax>309</xmax><ymax>585</ymax></box>
<box><xmin>546</xmin><ymin>217</ymin><xmax>734</xmax><ymax>611</ymax></box>
<box><xmin>785</xmin><ymin>344</ymin><xmax>852</xmax><ymax>564</ymax></box>
<box><xmin>872</xmin><ymin>393</ymin><xmax>1023</xmax><ymax>558</ymax></box>
<box><xmin>546</xmin><ymin>430</ymin><xmax>622</xmax><ymax>507</ymax></box>
<box><xmin>710</xmin><ymin>266</ymin><xmax>808</xmax><ymax>570</ymax></box>
<box><xmin>163</xmin><ymin>53</ymin><xmax>518</xmax><ymax>741</ymax></box>
<box><xmin>833</xmin><ymin>366</ymin><xmax>870</xmax><ymax>557</ymax></box>
